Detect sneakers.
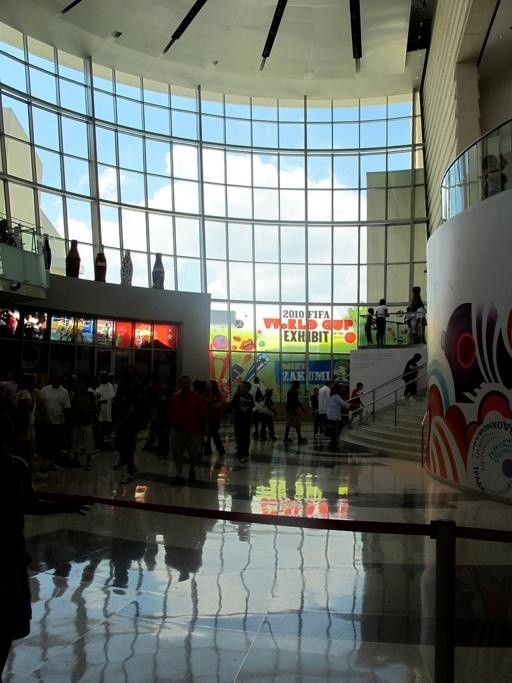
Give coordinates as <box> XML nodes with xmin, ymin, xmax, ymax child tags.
<box><xmin>201</xmin><ymin>453</ymin><xmax>250</xmax><ymax>464</ymax></box>
<box><xmin>120</xmin><ymin>474</ymin><xmax>133</xmax><ymax>484</ymax></box>
<box><xmin>114</xmin><ymin>459</ymin><xmax>127</xmax><ymax>470</ymax></box>
<box><xmin>284</xmin><ymin>438</ymin><xmax>307</xmax><ymax>442</ymax></box>
<box><xmin>399</xmin><ymin>395</ymin><xmax>417</xmax><ymax>402</ymax></box>
<box><xmin>189</xmin><ymin>470</ymin><xmax>196</xmax><ymax>486</ymax></box>
<box><xmin>252</xmin><ymin>431</ymin><xmax>277</xmax><ymax>442</ymax></box>
<box><xmin>171</xmin><ymin>475</ymin><xmax>184</xmax><ymax>486</ymax></box>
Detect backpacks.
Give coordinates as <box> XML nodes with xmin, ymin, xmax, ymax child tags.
<box><xmin>252</xmin><ymin>384</ymin><xmax>264</xmax><ymax>402</ymax></box>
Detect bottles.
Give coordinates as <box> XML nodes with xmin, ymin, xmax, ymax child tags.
<box><xmin>1</xmin><ymin>218</ymin><xmax>16</xmax><ymax>245</ymax></box>
<box><xmin>42</xmin><ymin>234</ymin><xmax>52</xmax><ymax>271</ymax></box>
<box><xmin>120</xmin><ymin>249</ymin><xmax>134</xmax><ymax>286</ymax></box>
<box><xmin>66</xmin><ymin>240</ymin><xmax>81</xmax><ymax>278</ymax></box>
<box><xmin>153</xmin><ymin>253</ymin><xmax>165</xmax><ymax>290</ymax></box>
<box><xmin>96</xmin><ymin>243</ymin><xmax>107</xmax><ymax>282</ymax></box>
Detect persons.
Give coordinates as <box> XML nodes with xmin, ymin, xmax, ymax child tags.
<box><xmin>0</xmin><ymin>303</ymin><xmax>112</xmax><ymax>344</ymax></box>
<box><xmin>416</xmin><ymin>304</ymin><xmax>428</xmax><ymax>344</ymax></box>
<box><xmin>401</xmin><ymin>353</ymin><xmax>425</xmax><ymax>402</ymax></box>
<box><xmin>0</xmin><ymin>369</ymin><xmax>369</xmax><ymax>487</ymax></box>
<box><xmin>375</xmin><ymin>299</ymin><xmax>389</xmax><ymax>346</ymax></box>
<box><xmin>481</xmin><ymin>154</ymin><xmax>509</xmax><ymax>201</ymax></box>
<box><xmin>0</xmin><ymin>398</ymin><xmax>97</xmax><ymax>683</ymax></box>
<box><xmin>364</xmin><ymin>308</ymin><xmax>376</xmax><ymax>345</ymax></box>
<box><xmin>403</xmin><ymin>307</ymin><xmax>417</xmax><ymax>345</ymax></box>
